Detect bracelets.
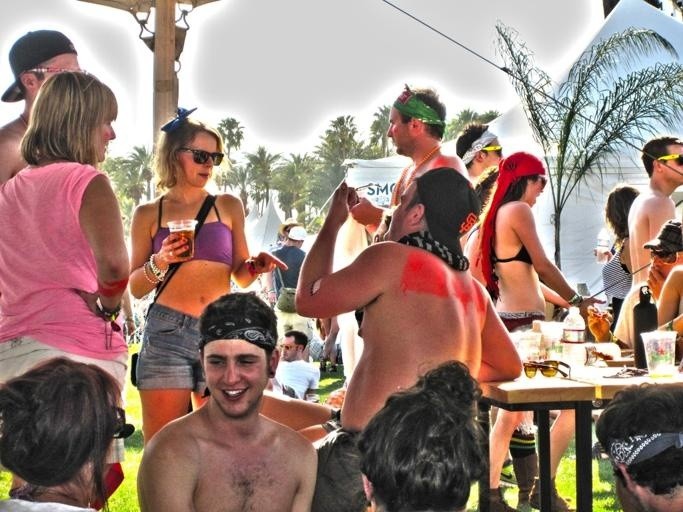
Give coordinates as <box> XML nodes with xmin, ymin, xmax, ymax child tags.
<box><xmin>143</xmin><ymin>254</ymin><xmax>168</xmax><ymax>284</ymax></box>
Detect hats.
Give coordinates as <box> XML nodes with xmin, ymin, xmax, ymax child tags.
<box><xmin>290</xmin><ymin>226</ymin><xmax>308</xmax><ymax>240</ymax></box>
<box><xmin>1</xmin><ymin>30</ymin><xmax>77</xmax><ymax>103</ymax></box>
<box><xmin>417</xmin><ymin>167</ymin><xmax>481</xmax><ymax>259</ymax></box>
<box><xmin>641</xmin><ymin>221</ymin><xmax>683</xmax><ymax>253</ymax></box>
<box><xmin>279</xmin><ymin>218</ymin><xmax>303</xmax><ymax>235</ymax></box>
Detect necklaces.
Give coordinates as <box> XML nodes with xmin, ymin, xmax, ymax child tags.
<box><xmin>392</xmin><ymin>145</ymin><xmax>444</xmax><ymax>207</ymax></box>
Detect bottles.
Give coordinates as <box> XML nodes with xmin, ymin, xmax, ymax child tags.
<box><xmin>563</xmin><ymin>307</ymin><xmax>586</xmax><ymax>367</ymax></box>
<box><xmin>633</xmin><ymin>286</ymin><xmax>658</xmax><ymax>368</ymax></box>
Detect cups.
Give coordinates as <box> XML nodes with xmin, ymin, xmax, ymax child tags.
<box><xmin>639</xmin><ymin>329</ymin><xmax>675</xmax><ymax>380</ymax></box>
<box><xmin>540</xmin><ymin>323</ymin><xmax>563</xmax><ymax>361</ymax></box>
<box><xmin>166</xmin><ymin>220</ymin><xmax>197</xmax><ymax>259</ymax></box>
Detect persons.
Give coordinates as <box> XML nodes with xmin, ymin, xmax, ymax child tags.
<box><xmin>318</xmin><ymin>311</ymin><xmax>343</xmax><ymax>373</ymax></box>
<box><xmin>603</xmin><ymin>188</ymin><xmax>640</xmax><ymax>333</ymax></box>
<box><xmin>351</xmin><ymin>86</ymin><xmax>472</xmax><ymax>339</ymax></box>
<box><xmin>265</xmin><ymin>331</ymin><xmax>319</xmax><ymax>403</ymax></box>
<box><xmin>272</xmin><ymin>227</ymin><xmax>314</xmax><ymax>344</ymax></box>
<box><xmin>267</xmin><ymin>224</ymin><xmax>303</xmax><ymax>307</ymax></box>
<box><xmin>457</xmin><ymin>124</ymin><xmax>536</xmax><ymax>455</ymax></box>
<box><xmin>0</xmin><ymin>73</ymin><xmax>133</xmax><ymax>510</ymax></box>
<box><xmin>612</xmin><ymin>220</ymin><xmax>682</xmax><ymax>348</ymax></box>
<box><xmin>628</xmin><ymin>137</ymin><xmax>682</xmax><ymax>289</ymax></box>
<box><xmin>130</xmin><ymin>107</ymin><xmax>288</xmax><ymax>455</ymax></box>
<box><xmin>654</xmin><ymin>265</ymin><xmax>683</xmax><ymax>367</ymax></box>
<box><xmin>1</xmin><ymin>29</ymin><xmax>82</xmax><ymax>188</ymax></box>
<box><xmin>291</xmin><ymin>167</ymin><xmax>523</xmax><ymax>510</ymax></box>
<box><xmin>476</xmin><ymin>150</ymin><xmax>606</xmax><ymax>510</ymax></box>
<box><xmin>596</xmin><ymin>383</ymin><xmax>681</xmax><ymax>509</ymax></box>
<box><xmin>136</xmin><ymin>295</ymin><xmax>318</xmax><ymax>512</ymax></box>
<box><xmin>356</xmin><ymin>360</ymin><xmax>484</xmax><ymax>510</ymax></box>
<box><xmin>0</xmin><ymin>356</ymin><xmax>124</xmax><ymax>510</ymax></box>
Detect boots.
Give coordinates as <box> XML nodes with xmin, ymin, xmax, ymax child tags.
<box><xmin>510</xmin><ymin>428</ymin><xmax>546</xmax><ymax>508</ymax></box>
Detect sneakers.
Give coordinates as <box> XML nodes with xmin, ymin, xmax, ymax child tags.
<box><xmin>530</xmin><ymin>478</ymin><xmax>571</xmax><ymax>511</ymax></box>
<box><xmin>326</xmin><ymin>374</ymin><xmax>339</xmax><ymax>380</ymax></box>
<box><xmin>487</xmin><ymin>488</ymin><xmax>517</xmax><ymax>511</ymax></box>
<box><xmin>320</xmin><ymin>358</ymin><xmax>326</xmax><ymax>370</ymax></box>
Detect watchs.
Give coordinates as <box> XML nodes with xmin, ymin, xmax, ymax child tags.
<box><xmin>244</xmin><ymin>257</ymin><xmax>259</xmax><ymax>280</ymax></box>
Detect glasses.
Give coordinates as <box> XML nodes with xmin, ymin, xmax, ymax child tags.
<box><xmin>282</xmin><ymin>345</ymin><xmax>300</xmax><ymax>349</ymax></box>
<box><xmin>22</xmin><ymin>68</ymin><xmax>87</xmax><ymax>76</ymax></box>
<box><xmin>95</xmin><ymin>407</ymin><xmax>125</xmax><ymax>436</ymax></box>
<box><xmin>656</xmin><ymin>155</ymin><xmax>683</xmax><ymax>165</ymax></box>
<box><xmin>174</xmin><ymin>147</ymin><xmax>224</xmax><ymax>166</ymax></box>
<box><xmin>525</xmin><ymin>361</ymin><xmax>570</xmax><ymax>379</ymax></box>
<box><xmin>476</xmin><ymin>145</ymin><xmax>503</xmax><ymax>157</ymax></box>
<box><xmin>535</xmin><ymin>175</ymin><xmax>546</xmax><ymax>187</ymax></box>
<box><xmin>647</xmin><ymin>248</ymin><xmax>680</xmax><ymax>264</ymax></box>
